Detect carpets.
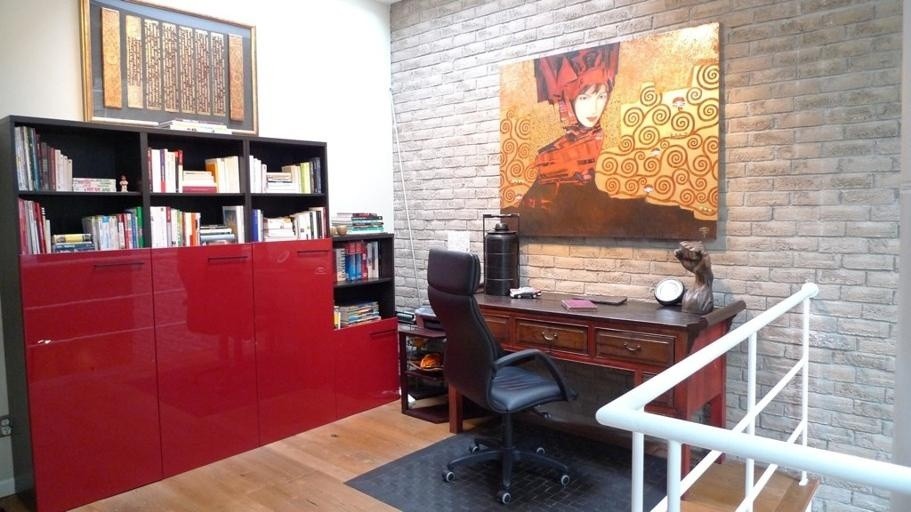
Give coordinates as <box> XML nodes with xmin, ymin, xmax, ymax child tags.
<box><xmin>343</xmin><ymin>412</ymin><xmax>683</xmax><ymax>512</ymax></box>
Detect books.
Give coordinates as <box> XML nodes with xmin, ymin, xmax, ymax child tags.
<box><xmin>15</xmin><ymin>126</ymin><xmax>117</xmax><ymax>193</ymax></box>
<box><xmin>249</xmin><ymin>155</ymin><xmax>321</xmax><ymax>194</ymax></box>
<box><xmin>18</xmin><ymin>198</ymin><xmax>51</xmax><ymax>255</ymax></box>
<box><xmin>92</xmin><ymin>117</ymin><xmax>257</xmax><ymax>136</ymax></box>
<box><xmin>251</xmin><ymin>207</ymin><xmax>327</xmax><ymax>242</ymax></box>
<box><xmin>150</xmin><ymin>206</ymin><xmax>245</xmax><ymax>249</ymax></box>
<box><xmin>148</xmin><ymin>147</ymin><xmax>241</xmax><ymax>194</ymax></box>
<box><xmin>51</xmin><ymin>233</ymin><xmax>95</xmax><ymax>253</ymax></box>
<box><xmin>330</xmin><ymin>211</ymin><xmax>386</xmax><ymax>329</ymax></box>
<box><xmin>82</xmin><ymin>207</ymin><xmax>143</xmax><ymax>250</ymax></box>
<box><xmin>561</xmin><ymin>299</ymin><xmax>599</xmax><ymax>312</ymax></box>
<box><xmin>589</xmin><ymin>293</ymin><xmax>628</xmax><ymax>304</ymax></box>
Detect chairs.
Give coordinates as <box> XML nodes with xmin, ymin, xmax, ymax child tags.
<box><xmin>423</xmin><ymin>247</ymin><xmax>578</xmax><ymax>504</ymax></box>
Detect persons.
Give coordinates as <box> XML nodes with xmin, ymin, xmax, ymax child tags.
<box><xmin>536</xmin><ymin>55</ymin><xmax>620</xmax><ymax>182</ymax></box>
<box><xmin>120</xmin><ymin>175</ymin><xmax>128</xmax><ymax>192</ymax></box>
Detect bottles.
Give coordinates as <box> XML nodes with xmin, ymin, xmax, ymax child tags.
<box><xmin>333</xmin><ymin>306</ymin><xmax>341</xmax><ymax>328</ymax></box>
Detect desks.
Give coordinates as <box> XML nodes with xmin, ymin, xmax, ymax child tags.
<box><xmin>446</xmin><ymin>282</ymin><xmax>746</xmax><ymax>488</ymax></box>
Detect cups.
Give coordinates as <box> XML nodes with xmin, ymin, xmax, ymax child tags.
<box><xmin>331</xmin><ymin>225</ymin><xmax>349</xmax><ymax>235</ymax></box>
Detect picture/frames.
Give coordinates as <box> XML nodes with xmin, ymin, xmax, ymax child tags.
<box><xmin>79</xmin><ymin>0</ymin><xmax>260</xmax><ymax>136</ymax></box>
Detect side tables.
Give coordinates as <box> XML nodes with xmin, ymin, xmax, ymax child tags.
<box><xmin>401</xmin><ymin>328</ymin><xmax>487</xmax><ymax>422</ymax></box>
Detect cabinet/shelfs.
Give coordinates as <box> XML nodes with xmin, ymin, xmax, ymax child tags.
<box><xmin>335</xmin><ymin>229</ymin><xmax>403</xmax><ymax>420</ymax></box>
<box><xmin>145</xmin><ymin>126</ymin><xmax>257</xmax><ymax>478</ymax></box>
<box><xmin>1</xmin><ymin>115</ymin><xmax>165</xmax><ymax>512</ymax></box>
<box><xmin>248</xmin><ymin>135</ymin><xmax>342</xmax><ymax>448</ymax></box>
